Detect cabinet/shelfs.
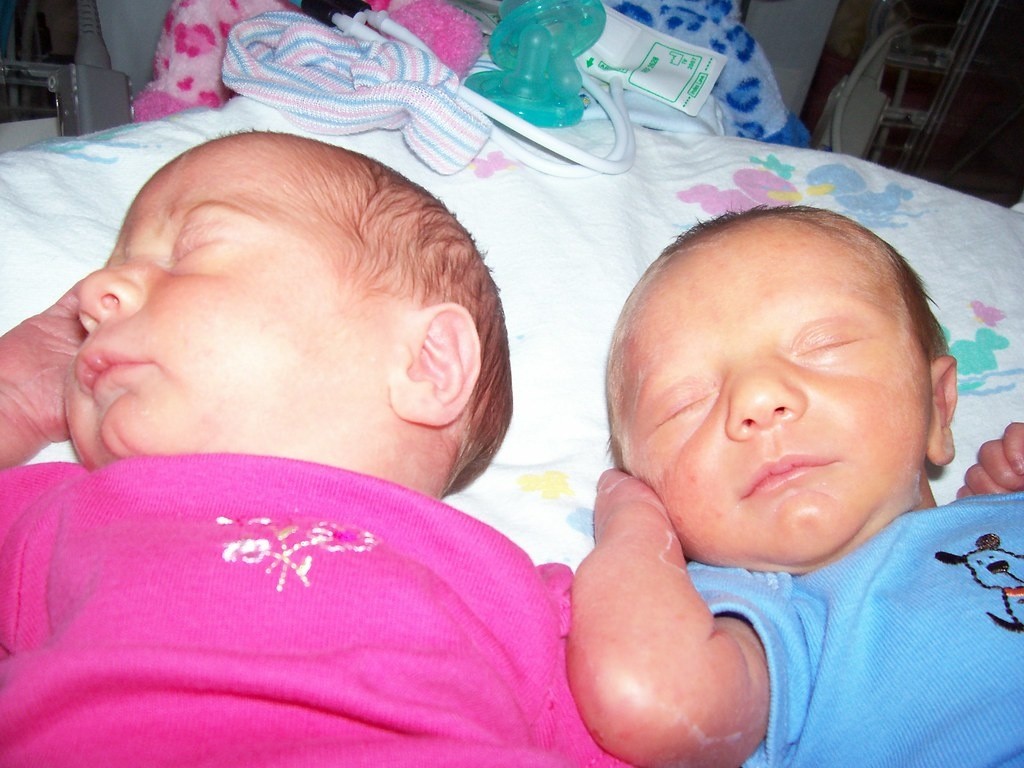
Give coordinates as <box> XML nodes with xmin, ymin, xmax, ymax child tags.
<box><xmin>793</xmin><ymin>0</ymin><xmax>1024</xmax><ymax>207</ymax></box>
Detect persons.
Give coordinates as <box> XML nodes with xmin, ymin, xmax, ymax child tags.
<box><xmin>563</xmin><ymin>200</ymin><xmax>1024</xmax><ymax>768</ymax></box>
<box><xmin>0</xmin><ymin>128</ymin><xmax>633</xmax><ymax>768</ymax></box>
